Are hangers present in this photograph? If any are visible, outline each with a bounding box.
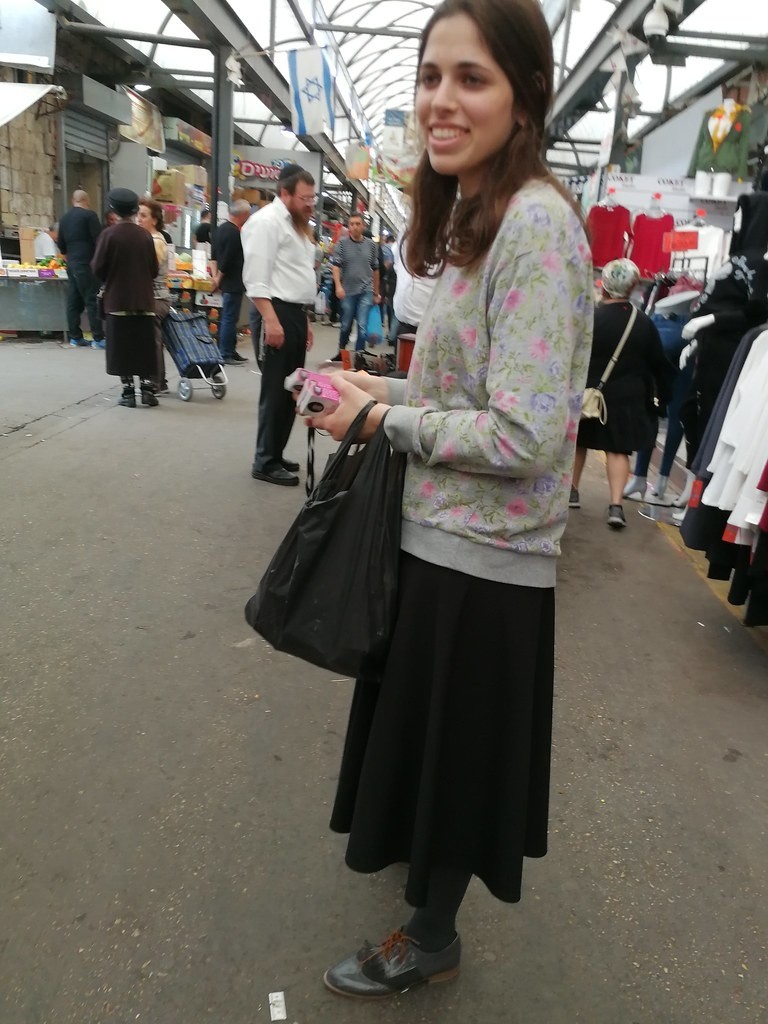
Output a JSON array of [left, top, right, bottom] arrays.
[[691, 215, 710, 227], [643, 197, 667, 216], [598, 192, 618, 207]]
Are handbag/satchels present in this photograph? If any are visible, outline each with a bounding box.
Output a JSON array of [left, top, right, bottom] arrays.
[[95, 297, 105, 321], [365, 304, 382, 344], [580, 388, 607, 426], [244, 400, 407, 686]]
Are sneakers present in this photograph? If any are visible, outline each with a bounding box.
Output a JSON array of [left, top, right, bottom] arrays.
[[70, 337, 90, 346], [567, 490, 581, 507], [608, 506, 627, 528], [92, 339, 106, 349]]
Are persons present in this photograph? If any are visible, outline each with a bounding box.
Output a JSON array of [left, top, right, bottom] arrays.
[[391, 235, 449, 365], [290, 0, 594, 1000], [33, 222, 61, 265], [104, 209, 119, 226], [321, 242, 342, 327], [568, 257, 669, 530], [211, 199, 252, 365], [240, 163, 316, 486], [362, 231, 397, 331], [89, 187, 160, 407], [308, 222, 324, 295], [622, 289, 700, 502], [134, 193, 170, 396], [326, 211, 381, 363], [57, 189, 107, 350], [193, 210, 212, 260], [672, 191, 768, 522]]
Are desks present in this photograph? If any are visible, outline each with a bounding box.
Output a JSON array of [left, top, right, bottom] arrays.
[[0, 276, 70, 343]]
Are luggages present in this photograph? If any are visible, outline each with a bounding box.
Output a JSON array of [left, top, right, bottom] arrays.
[[159, 306, 228, 401]]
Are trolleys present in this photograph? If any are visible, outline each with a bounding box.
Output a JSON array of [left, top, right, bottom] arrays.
[[162, 305, 228, 401]]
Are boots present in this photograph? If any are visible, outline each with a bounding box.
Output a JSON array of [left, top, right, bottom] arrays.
[[141, 383, 158, 406], [118, 387, 136, 407]]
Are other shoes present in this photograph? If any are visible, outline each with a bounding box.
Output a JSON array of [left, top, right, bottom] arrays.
[[332, 321, 342, 327], [223, 356, 241, 365], [321, 320, 332, 326], [140, 382, 169, 396], [330, 354, 343, 361], [232, 351, 244, 361]]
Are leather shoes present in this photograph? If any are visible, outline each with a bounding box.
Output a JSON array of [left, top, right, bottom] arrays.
[[251, 467, 298, 486], [282, 458, 299, 471], [324, 925, 461, 1000]]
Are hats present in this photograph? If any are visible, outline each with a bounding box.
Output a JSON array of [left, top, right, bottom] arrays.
[[601, 258, 640, 300]]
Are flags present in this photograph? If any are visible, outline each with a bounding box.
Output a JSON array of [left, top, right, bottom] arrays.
[[289, 46, 334, 135]]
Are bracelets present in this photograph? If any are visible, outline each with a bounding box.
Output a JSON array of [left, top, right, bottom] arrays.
[[373, 292, 379, 296]]
